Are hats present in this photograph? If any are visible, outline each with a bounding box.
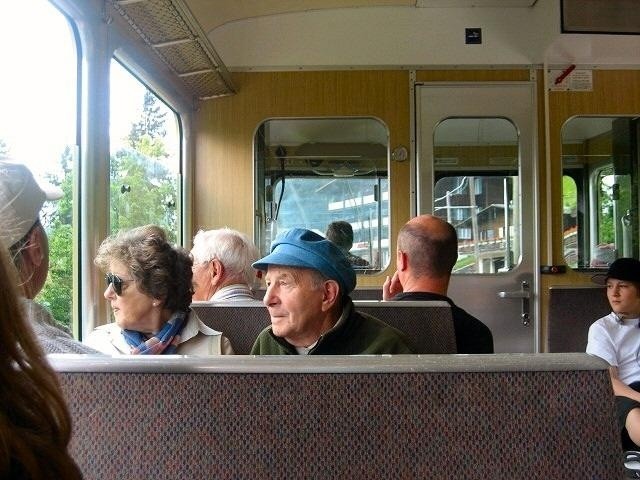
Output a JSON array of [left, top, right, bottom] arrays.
[[0, 163, 64, 247], [592, 258, 639, 285], [252, 228, 357, 294]]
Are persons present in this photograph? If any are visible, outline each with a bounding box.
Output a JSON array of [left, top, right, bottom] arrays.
[[381, 214, 496, 355], [327, 221, 368, 268], [189, 227, 264, 305], [252, 228, 417, 355], [0, 229, 84, 480], [0, 159, 103, 356], [85, 225, 234, 355], [587, 256, 640, 448]]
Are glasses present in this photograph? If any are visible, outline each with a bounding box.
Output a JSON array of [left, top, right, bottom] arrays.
[[105, 273, 135, 296]]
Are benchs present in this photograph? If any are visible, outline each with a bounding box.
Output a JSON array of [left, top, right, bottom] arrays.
[[41, 286, 640, 480]]
